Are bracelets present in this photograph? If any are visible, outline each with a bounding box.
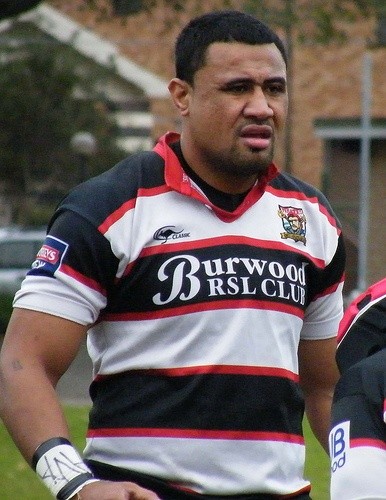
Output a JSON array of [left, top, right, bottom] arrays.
[[31, 437, 100, 500]]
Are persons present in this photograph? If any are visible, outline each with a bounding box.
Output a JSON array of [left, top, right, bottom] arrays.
[[0, 11, 386, 500]]
